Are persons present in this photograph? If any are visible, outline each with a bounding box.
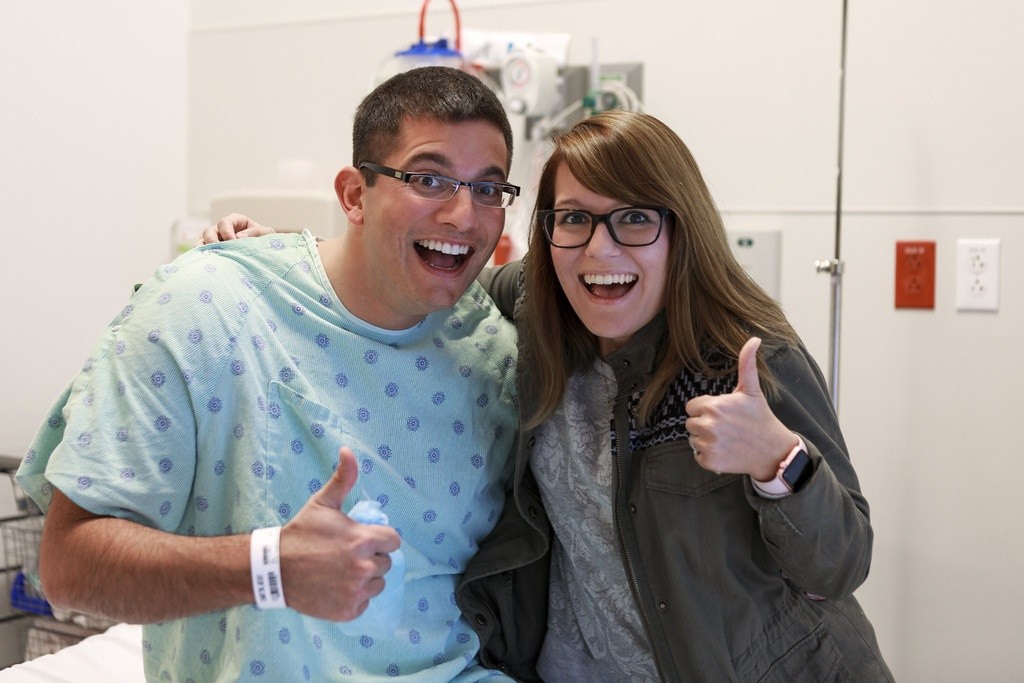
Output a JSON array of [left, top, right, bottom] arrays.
[[15, 69, 529, 683], [194, 111, 898, 683]]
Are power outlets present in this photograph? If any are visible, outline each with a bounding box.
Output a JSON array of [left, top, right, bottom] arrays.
[[959, 238, 1002, 313]]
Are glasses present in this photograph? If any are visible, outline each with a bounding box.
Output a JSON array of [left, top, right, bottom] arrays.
[[541, 205, 672, 250], [360, 160, 520, 211]]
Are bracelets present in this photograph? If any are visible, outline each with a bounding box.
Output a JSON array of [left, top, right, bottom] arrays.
[[250, 526, 288, 611]]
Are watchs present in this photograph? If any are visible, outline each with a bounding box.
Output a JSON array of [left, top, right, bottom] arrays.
[[749, 434, 812, 496]]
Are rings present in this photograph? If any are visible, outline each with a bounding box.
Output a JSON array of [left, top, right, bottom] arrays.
[[690, 438, 699, 455]]
